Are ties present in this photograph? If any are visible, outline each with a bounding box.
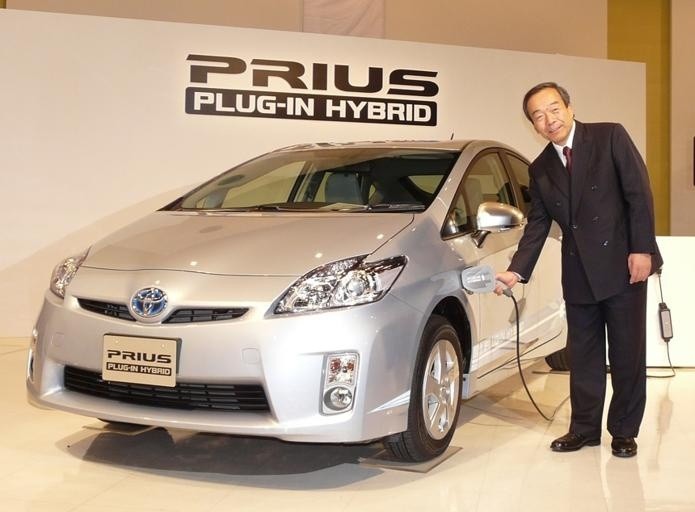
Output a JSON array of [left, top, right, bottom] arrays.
[[563, 146, 572, 175]]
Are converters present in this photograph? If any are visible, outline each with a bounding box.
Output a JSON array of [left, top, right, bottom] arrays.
[[658, 303, 673, 342]]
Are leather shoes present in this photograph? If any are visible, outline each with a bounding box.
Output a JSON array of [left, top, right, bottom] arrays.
[[550, 432, 601, 452], [611, 436, 638, 457]]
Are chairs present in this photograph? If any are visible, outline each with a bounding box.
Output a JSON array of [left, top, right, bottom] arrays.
[[324, 171, 364, 205], [367, 169, 485, 239]]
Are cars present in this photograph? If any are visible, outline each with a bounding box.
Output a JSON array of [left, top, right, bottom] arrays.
[[23, 138, 568, 464]]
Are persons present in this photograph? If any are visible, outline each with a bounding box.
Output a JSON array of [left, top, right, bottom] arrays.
[[488, 79, 666, 457]]
